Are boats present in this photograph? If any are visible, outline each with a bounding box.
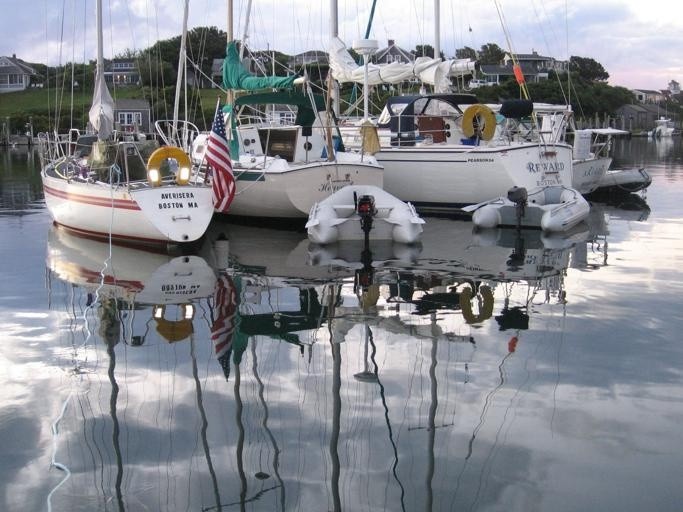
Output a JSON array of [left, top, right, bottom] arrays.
[[606, 164, 653, 195], [463, 183, 590, 235], [303, 185, 426, 246], [470, 221, 594, 256], [304, 242, 423, 268], [646, 117, 674, 136]]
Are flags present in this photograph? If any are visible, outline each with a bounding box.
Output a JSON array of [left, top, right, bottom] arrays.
[[205, 98, 236, 213], [210, 269, 236, 383]]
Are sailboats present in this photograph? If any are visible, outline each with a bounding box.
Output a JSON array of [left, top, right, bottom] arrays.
[[575, 146, 613, 190], [217, 210, 388, 509], [37, 1, 217, 255], [373, 0, 576, 216], [45, 221, 220, 508], [388, 214, 606, 512], [213, 1, 385, 222]]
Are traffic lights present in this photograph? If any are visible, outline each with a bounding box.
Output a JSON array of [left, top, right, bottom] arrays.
[[358, 196, 372, 217], [358, 269, 370, 285]]
